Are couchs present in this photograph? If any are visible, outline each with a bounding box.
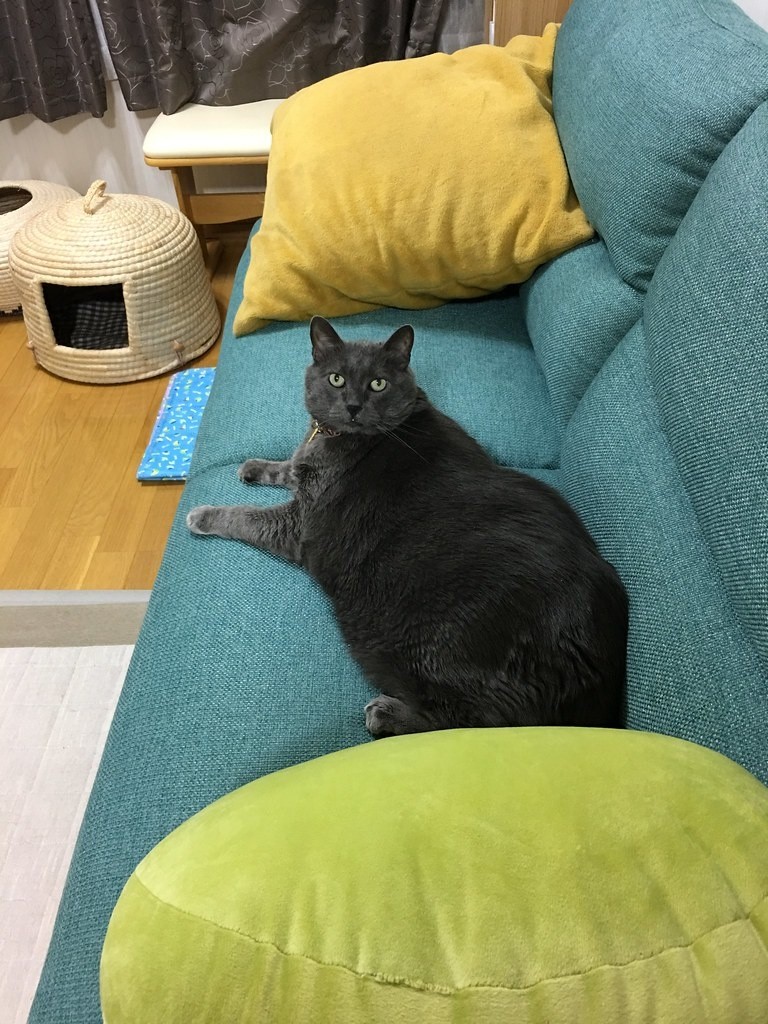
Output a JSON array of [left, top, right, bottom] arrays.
[[21, 0, 767, 1023]]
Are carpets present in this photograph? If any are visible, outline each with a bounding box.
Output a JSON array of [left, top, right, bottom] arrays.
[[2, 591, 152, 1024]]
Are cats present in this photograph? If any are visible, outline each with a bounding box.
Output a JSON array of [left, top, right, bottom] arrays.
[[185, 317, 629, 738]]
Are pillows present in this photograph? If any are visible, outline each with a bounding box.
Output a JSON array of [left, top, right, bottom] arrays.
[[230, 22, 597, 336], [94, 726, 767, 1021]]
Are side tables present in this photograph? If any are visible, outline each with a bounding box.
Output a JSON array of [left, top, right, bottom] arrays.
[[142, 97, 275, 264]]
